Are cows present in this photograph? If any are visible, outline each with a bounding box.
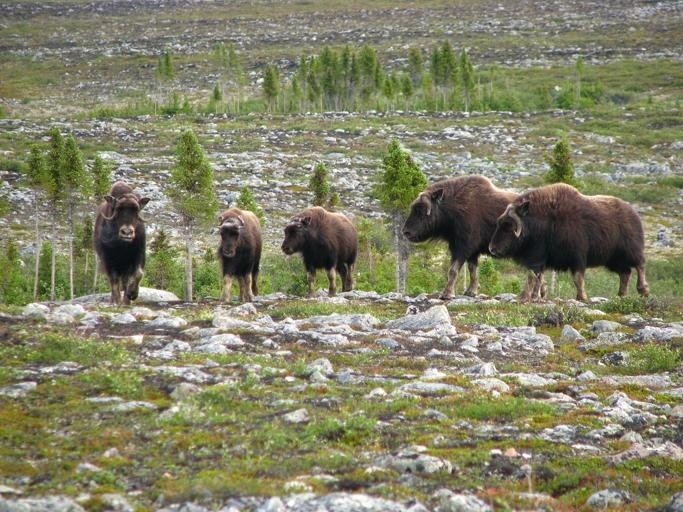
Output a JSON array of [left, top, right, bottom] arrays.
[[280, 206, 359, 300], [489, 182, 651, 302], [92, 181, 150, 312], [401, 173, 547, 302], [216, 207, 263, 305]]
